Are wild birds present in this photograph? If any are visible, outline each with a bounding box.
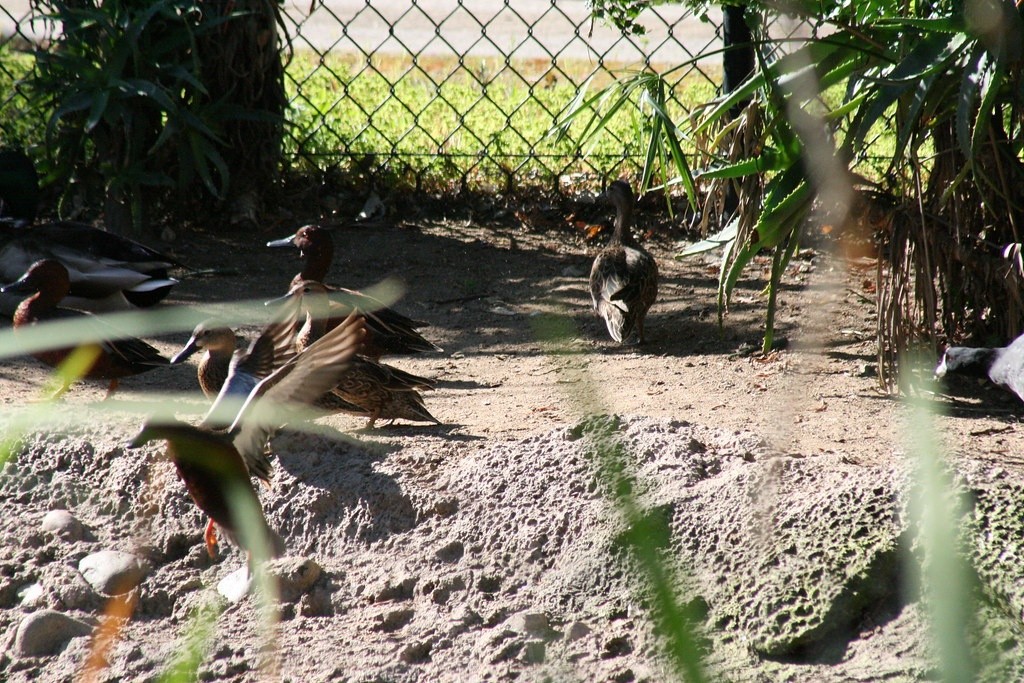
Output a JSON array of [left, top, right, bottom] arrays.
[[124, 224, 443, 582], [932, 332, 1024, 404], [0, 217, 181, 403], [587, 180, 658, 346]]
